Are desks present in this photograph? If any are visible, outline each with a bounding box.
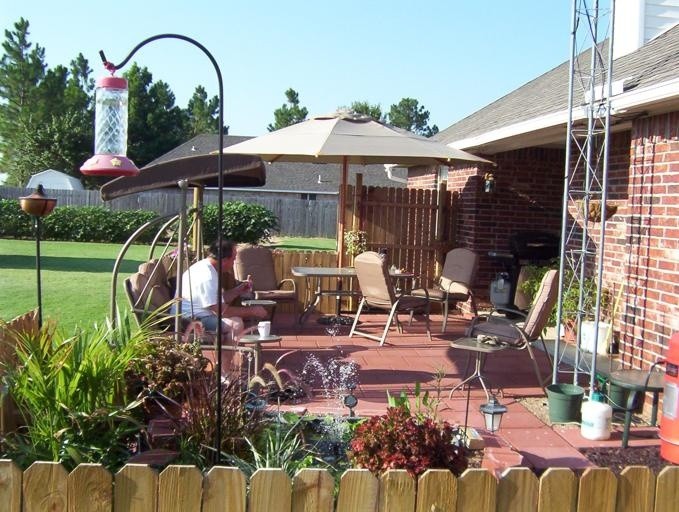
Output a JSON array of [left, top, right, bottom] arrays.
[[609, 359, 666, 451], [292, 264, 414, 328], [447, 336, 508, 402]]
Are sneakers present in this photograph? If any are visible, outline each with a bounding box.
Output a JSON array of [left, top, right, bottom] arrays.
[[220, 373, 246, 387]]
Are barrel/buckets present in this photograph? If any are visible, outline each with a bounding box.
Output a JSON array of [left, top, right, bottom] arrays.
[[580, 399, 613, 441], [578, 319, 611, 356], [659, 315, 679, 465], [543, 382, 586, 423], [243, 391, 267, 417], [605, 384, 626, 409]]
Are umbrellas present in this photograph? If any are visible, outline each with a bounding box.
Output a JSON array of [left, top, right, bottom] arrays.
[[209, 109, 497, 315]]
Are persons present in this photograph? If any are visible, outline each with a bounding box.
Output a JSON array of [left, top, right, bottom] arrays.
[[170, 239, 267, 386]]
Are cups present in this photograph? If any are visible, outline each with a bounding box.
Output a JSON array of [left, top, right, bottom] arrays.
[[257, 321, 271, 337]]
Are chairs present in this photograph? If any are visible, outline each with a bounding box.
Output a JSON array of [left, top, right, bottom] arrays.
[[409, 248, 480, 331], [462, 269, 558, 396], [348, 252, 433, 347], [130, 246, 298, 382]]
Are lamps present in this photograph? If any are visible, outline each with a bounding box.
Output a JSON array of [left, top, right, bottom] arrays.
[[478, 396, 507, 433]]
